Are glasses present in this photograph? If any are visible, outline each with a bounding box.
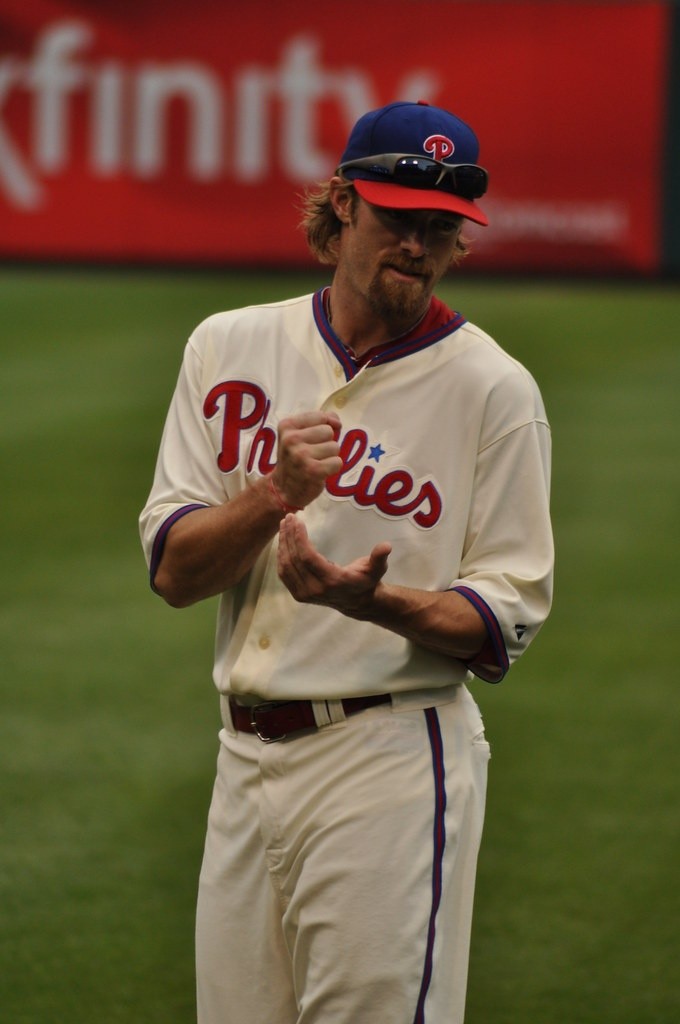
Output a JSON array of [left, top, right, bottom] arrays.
[[335, 154, 489, 200]]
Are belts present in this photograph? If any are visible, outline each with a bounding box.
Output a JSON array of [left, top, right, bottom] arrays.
[[229, 694, 392, 745]]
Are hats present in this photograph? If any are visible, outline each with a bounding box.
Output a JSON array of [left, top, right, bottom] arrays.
[[340, 99, 491, 227]]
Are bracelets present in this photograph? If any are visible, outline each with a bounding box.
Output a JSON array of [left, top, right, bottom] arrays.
[[269, 471, 304, 514]]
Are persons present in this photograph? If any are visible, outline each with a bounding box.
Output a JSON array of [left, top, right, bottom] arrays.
[[136, 98, 557, 1024]]
